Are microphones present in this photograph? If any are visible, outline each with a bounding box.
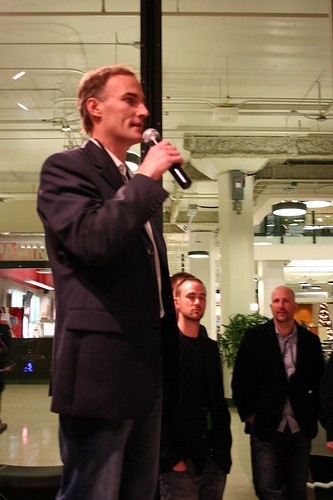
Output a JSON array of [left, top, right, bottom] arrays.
[[142, 128, 192, 189]]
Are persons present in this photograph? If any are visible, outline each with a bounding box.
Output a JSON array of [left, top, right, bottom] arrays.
[[37, 65, 186, 498], [168, 272, 193, 312], [320, 352, 333, 453], [156, 276, 234, 500], [230, 286, 326, 500], [1, 333, 9, 434]]
